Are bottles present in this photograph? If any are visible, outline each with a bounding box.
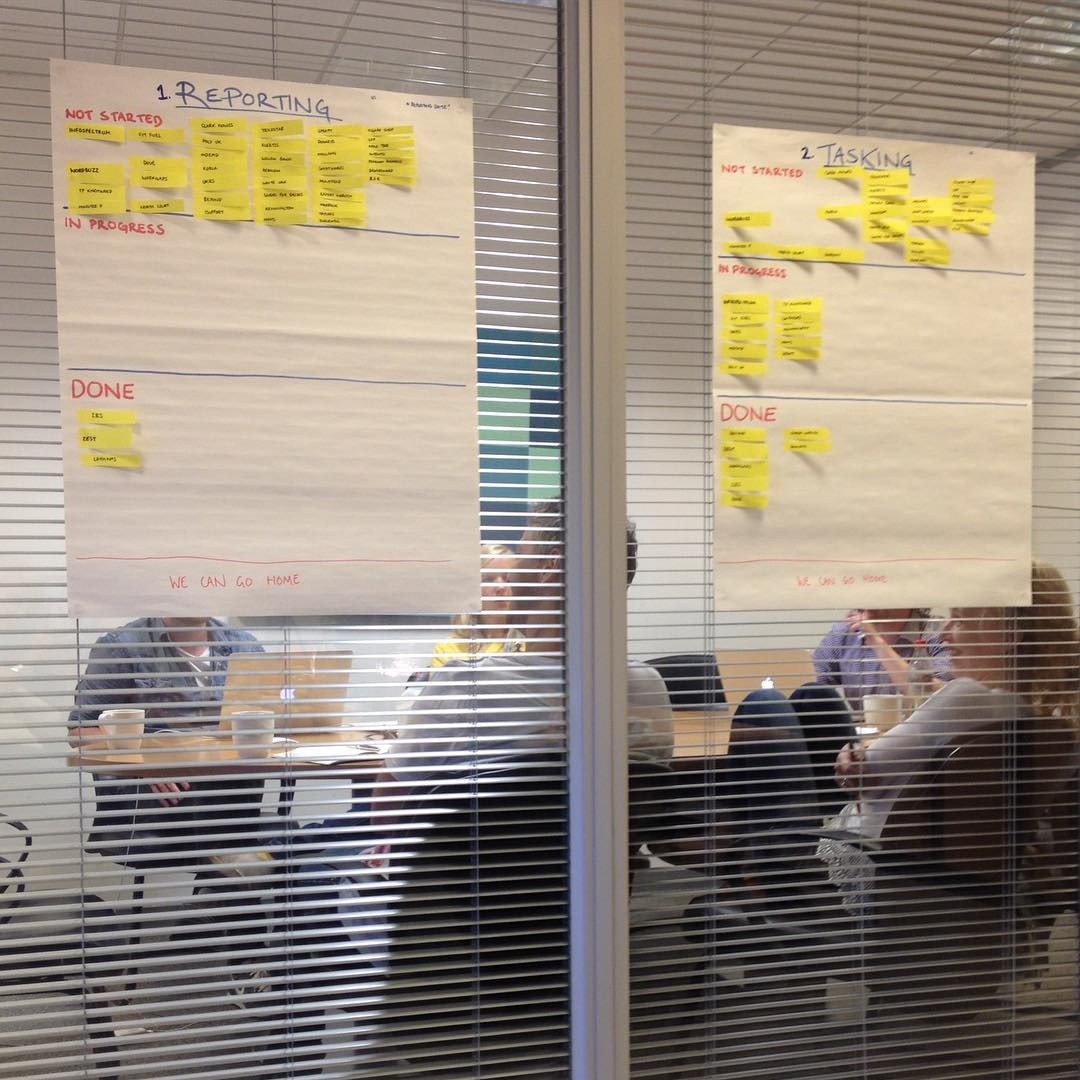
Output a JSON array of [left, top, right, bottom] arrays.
[[907, 640, 933, 716]]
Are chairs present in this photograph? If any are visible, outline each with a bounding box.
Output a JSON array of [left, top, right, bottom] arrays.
[[677, 714, 1080, 1079], [0, 813, 128, 1080], [644, 656, 728, 869], [311, 759, 715, 1080]]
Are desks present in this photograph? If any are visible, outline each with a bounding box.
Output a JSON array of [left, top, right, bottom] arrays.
[[68, 713, 879, 1006]]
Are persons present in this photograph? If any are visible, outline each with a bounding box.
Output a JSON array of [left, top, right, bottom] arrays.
[[66, 493, 1079, 1030]]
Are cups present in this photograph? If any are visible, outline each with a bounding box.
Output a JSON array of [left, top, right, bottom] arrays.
[[863, 695, 903, 731], [98, 709, 146, 750], [232, 710, 274, 760]]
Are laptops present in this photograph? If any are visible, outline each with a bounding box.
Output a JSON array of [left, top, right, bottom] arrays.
[[188, 650, 352, 738], [713, 648, 818, 715]]
